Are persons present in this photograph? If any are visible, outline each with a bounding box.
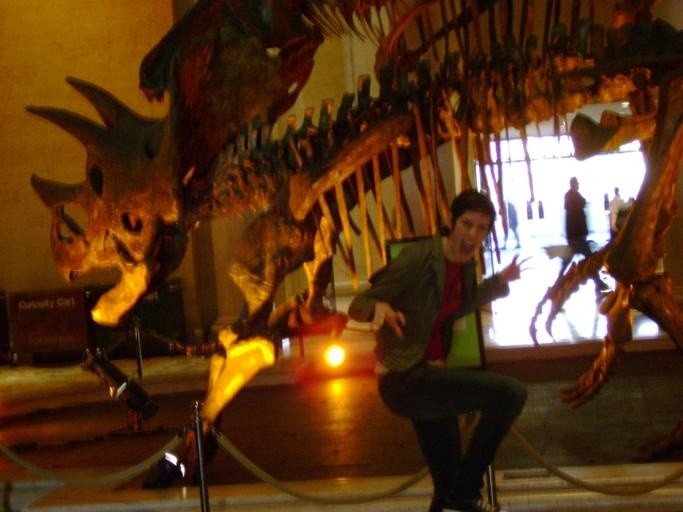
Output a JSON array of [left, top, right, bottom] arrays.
[[348, 186, 532, 512], [495, 193, 520, 250], [608, 186, 624, 228], [562, 175, 589, 244]]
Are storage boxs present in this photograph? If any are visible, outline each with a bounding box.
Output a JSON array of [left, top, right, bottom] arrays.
[[85, 277, 185, 359], [7, 280, 97, 365]]
